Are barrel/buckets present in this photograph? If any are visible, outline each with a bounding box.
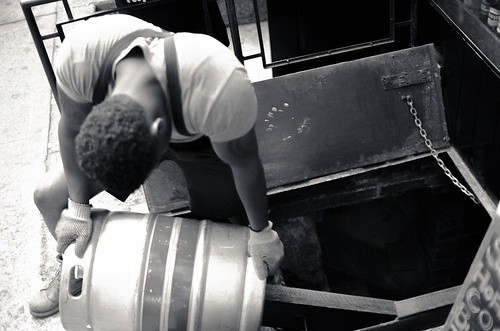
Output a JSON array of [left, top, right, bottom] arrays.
[[59, 208, 267, 331]]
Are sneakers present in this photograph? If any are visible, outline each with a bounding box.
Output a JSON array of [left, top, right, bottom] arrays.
[[29, 254, 82, 317]]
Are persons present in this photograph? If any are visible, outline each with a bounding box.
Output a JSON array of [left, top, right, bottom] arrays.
[[29, 14, 284, 317]]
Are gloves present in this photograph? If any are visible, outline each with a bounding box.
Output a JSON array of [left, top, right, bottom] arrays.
[[248, 221, 285, 280], [54, 198, 93, 258]]
